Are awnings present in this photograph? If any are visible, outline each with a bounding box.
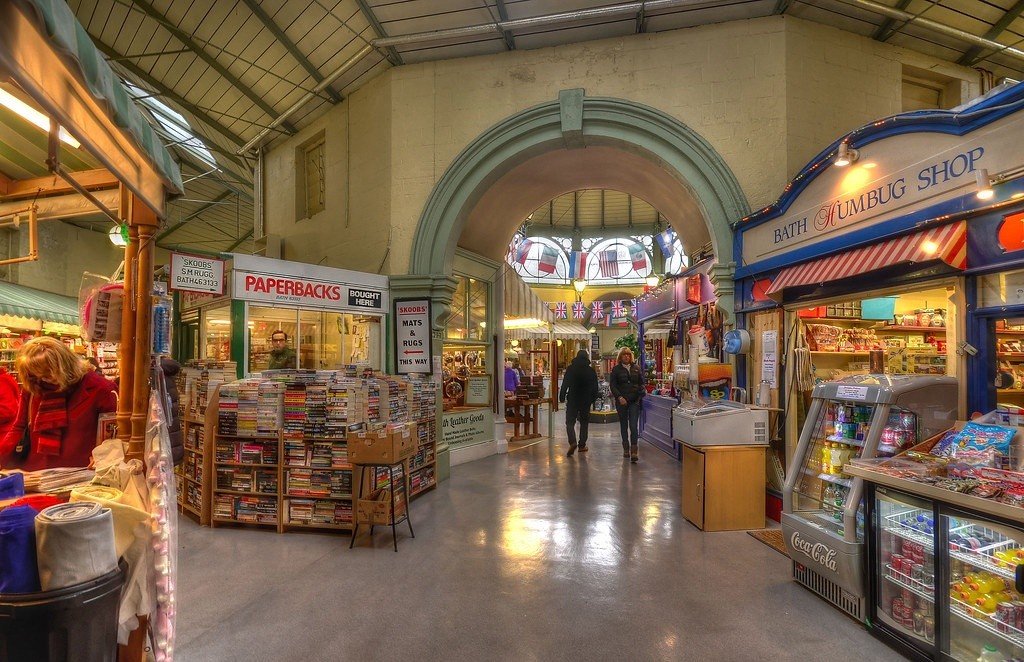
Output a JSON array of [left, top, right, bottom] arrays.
[[503, 321, 592, 385], [0, 280, 79, 326]]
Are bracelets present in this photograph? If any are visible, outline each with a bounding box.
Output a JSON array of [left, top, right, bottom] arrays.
[[620, 397, 623, 399]]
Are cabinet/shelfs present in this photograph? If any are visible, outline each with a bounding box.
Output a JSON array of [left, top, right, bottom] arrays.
[[681, 444, 766, 531], [1, 323, 488, 533], [878, 502, 1024, 662], [799, 318, 1024, 393]]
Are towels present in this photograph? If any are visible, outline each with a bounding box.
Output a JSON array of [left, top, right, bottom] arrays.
[[792, 344, 815, 393]]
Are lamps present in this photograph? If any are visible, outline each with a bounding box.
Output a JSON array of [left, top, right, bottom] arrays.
[[0, 88, 85, 151], [835, 144, 860, 166], [108, 223, 130, 249], [976, 169, 999, 200]]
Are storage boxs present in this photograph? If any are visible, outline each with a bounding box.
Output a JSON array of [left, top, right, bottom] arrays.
[[884, 336, 946, 353], [357, 489, 406, 526], [827, 300, 861, 319], [860, 296, 901, 319], [798, 306, 827, 318], [347, 421, 418, 464]]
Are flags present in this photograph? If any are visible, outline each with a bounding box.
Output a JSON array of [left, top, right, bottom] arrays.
[[572, 302, 584, 318], [611, 300, 623, 318], [592, 301, 604, 318], [569, 252, 587, 278], [538, 246, 559, 274], [599, 250, 619, 277], [629, 242, 646, 271], [654, 228, 674, 259], [516, 237, 532, 264], [631, 299, 637, 316], [556, 302, 566, 319]]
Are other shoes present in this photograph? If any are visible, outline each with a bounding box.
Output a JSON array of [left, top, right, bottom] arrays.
[[565, 442, 578, 456], [577, 446, 589, 452]]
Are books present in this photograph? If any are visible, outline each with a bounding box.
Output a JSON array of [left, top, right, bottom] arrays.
[[179, 360, 436, 525]]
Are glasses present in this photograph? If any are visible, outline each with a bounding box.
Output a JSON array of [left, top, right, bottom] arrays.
[[273, 339, 286, 343]]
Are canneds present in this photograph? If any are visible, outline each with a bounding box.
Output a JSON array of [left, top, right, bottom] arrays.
[[995, 600, 1024, 634], [889, 539, 973, 642], [880, 409, 917, 450]]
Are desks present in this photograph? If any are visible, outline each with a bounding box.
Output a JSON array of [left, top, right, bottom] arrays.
[[506, 398, 552, 442]]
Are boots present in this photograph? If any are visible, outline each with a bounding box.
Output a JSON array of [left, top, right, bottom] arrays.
[[631, 445, 640, 462], [621, 441, 631, 458]]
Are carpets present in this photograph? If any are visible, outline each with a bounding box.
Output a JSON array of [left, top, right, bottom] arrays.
[[746, 528, 788, 557]]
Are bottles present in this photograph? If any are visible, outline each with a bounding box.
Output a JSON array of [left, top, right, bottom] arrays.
[[903, 514, 1024, 619], [822, 441, 865, 532], [977, 643, 1005, 662]]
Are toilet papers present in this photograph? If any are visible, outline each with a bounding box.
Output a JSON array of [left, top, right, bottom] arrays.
[[721, 329, 751, 355]]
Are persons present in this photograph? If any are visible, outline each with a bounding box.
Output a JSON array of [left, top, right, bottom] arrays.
[[268, 331, 297, 369], [610, 347, 646, 462], [151, 358, 184, 467], [0, 351, 20, 444], [506, 361, 524, 416], [560, 350, 598, 455], [0, 337, 119, 471]]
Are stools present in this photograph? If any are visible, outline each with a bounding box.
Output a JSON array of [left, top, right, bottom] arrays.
[[350, 458, 417, 551]]
